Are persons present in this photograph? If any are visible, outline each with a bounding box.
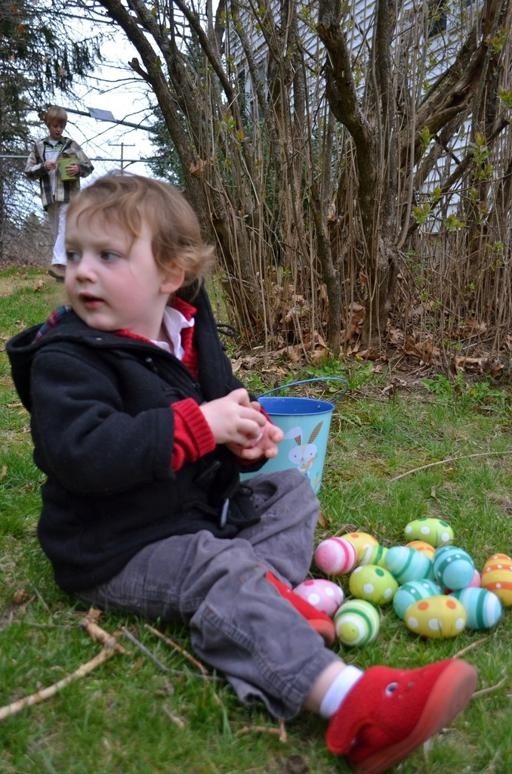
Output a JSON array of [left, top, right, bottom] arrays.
[[25, 106, 94, 282], [5, 166, 481, 774]]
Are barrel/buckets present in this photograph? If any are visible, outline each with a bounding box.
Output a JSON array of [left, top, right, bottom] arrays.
[[239, 378, 351, 501], [58, 158, 78, 180]]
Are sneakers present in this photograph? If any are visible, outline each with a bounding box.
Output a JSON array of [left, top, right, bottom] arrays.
[[48, 265, 64, 280], [265, 572, 336, 646], [326, 658, 477, 773]]
